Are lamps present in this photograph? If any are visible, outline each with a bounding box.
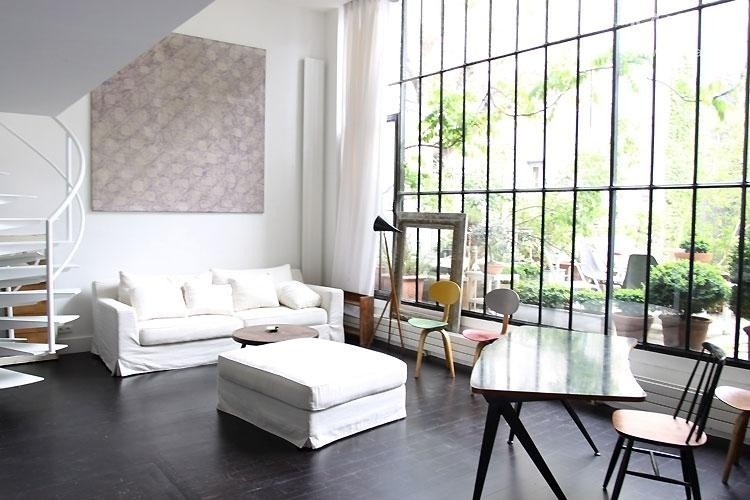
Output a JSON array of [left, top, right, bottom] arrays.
[[366, 217, 407, 357]]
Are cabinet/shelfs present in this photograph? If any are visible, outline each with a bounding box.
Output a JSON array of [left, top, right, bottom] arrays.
[[343, 291, 375, 348]]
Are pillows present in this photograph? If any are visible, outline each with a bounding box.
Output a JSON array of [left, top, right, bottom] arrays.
[[181, 283, 235, 316], [117, 270, 168, 307], [279, 279, 320, 309], [228, 276, 279, 311], [130, 283, 187, 320], [160, 270, 212, 295], [208, 268, 248, 285], [249, 263, 292, 292]]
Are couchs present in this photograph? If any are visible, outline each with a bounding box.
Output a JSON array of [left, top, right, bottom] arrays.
[[92, 264, 344, 378]]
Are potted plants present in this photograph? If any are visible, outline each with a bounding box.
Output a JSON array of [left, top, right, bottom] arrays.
[[540, 287, 571, 328], [572, 288, 606, 333], [512, 284, 540, 324], [674, 239, 712, 263], [646, 258, 732, 352], [609, 288, 654, 339], [728, 218, 749, 363]]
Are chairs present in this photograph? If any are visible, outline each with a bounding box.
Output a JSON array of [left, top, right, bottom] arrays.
[[408, 280, 460, 378], [462, 289, 520, 396], [715, 326, 750, 485], [602, 342, 726, 500]]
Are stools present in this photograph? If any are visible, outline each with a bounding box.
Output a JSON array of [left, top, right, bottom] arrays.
[[219, 337, 407, 455]]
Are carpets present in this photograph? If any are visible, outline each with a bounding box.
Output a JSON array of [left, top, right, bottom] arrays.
[[0, 367, 44, 390]]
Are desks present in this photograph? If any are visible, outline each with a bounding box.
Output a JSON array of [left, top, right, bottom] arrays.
[[470, 326, 647, 500]]
